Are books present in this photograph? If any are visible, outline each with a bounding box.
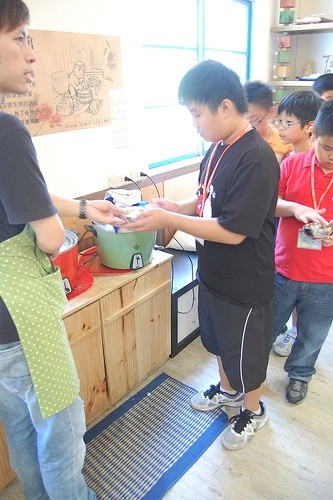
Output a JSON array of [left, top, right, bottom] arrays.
[[298, 16, 333, 25]]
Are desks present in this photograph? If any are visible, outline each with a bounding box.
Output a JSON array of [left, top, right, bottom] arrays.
[[155, 248, 202, 359], [0, 249, 175, 490]]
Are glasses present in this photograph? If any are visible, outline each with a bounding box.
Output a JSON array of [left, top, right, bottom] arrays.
[[272, 115, 310, 129], [247, 107, 271, 127]]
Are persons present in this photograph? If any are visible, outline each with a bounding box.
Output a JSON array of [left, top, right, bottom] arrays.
[[123, 60, 333, 452], [1, 0, 126, 499]]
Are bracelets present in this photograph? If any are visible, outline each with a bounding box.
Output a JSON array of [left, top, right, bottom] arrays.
[[79, 198, 87, 219]]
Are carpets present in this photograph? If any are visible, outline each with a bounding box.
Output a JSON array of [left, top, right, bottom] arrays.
[[82, 372, 230, 500]]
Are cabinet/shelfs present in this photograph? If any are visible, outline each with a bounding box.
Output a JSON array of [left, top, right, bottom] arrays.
[[268, 22, 333, 87]]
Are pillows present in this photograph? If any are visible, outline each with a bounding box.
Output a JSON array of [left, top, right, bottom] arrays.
[[165, 194, 211, 252]]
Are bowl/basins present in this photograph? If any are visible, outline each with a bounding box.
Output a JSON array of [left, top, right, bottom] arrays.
[[303, 222, 333, 240], [122, 206, 144, 222]]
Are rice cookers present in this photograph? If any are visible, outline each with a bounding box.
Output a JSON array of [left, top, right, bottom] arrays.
[[84, 220, 157, 269], [52, 229, 78, 294]]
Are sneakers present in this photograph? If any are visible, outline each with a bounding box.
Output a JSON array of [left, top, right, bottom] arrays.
[[285, 378, 308, 404], [190, 381, 245, 411], [221, 401, 268, 451], [274, 327, 298, 357]]
